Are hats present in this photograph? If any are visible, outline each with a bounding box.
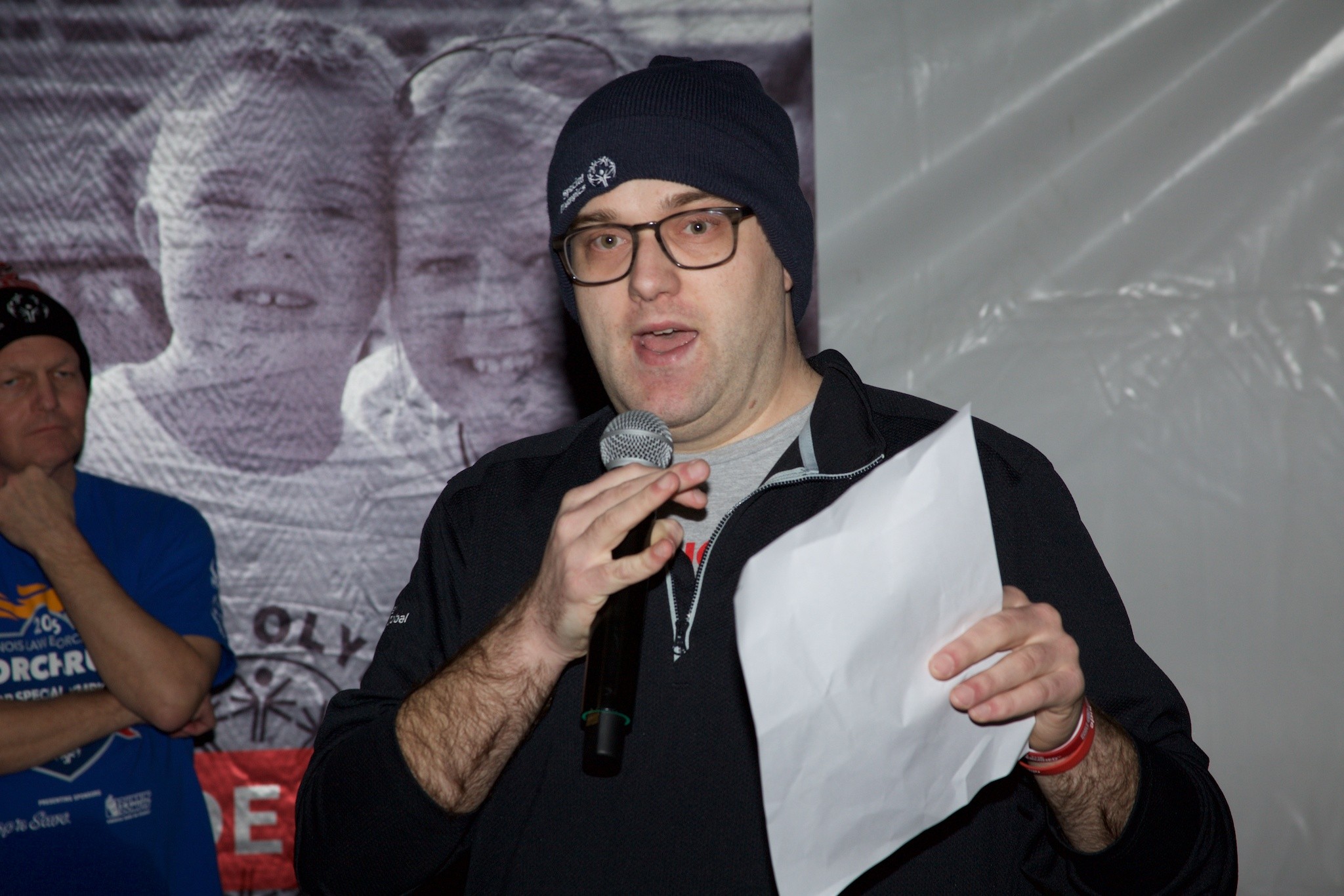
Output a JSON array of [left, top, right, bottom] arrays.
[[0, 274, 91, 390], [545, 54, 814, 327]]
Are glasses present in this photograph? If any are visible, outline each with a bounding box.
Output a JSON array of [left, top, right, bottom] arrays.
[[552, 205, 754, 287], [393, 31, 616, 117]]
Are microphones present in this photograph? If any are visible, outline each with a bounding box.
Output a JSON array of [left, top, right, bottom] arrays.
[[579, 410, 673, 775]]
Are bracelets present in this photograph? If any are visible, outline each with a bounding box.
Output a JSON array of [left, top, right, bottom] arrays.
[[1018, 697, 1097, 775], [1025, 701, 1091, 762]]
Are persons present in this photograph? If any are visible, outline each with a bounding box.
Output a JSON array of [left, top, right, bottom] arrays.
[[342, 31, 637, 481], [293, 55, 1240, 896], [0, 280, 239, 896], [76, 12, 445, 752]]
